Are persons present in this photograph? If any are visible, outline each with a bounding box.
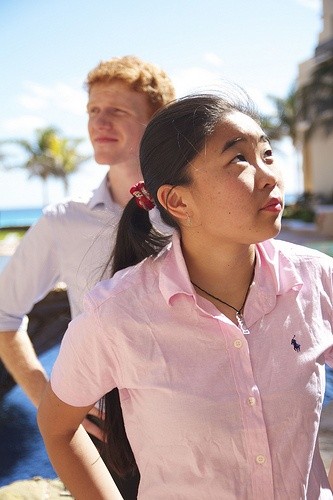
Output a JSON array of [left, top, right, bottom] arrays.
[[32, 93, 333, 500], [0, 57, 176, 500]]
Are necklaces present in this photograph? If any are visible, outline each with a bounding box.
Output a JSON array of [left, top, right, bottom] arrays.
[[190, 251, 256, 335]]
